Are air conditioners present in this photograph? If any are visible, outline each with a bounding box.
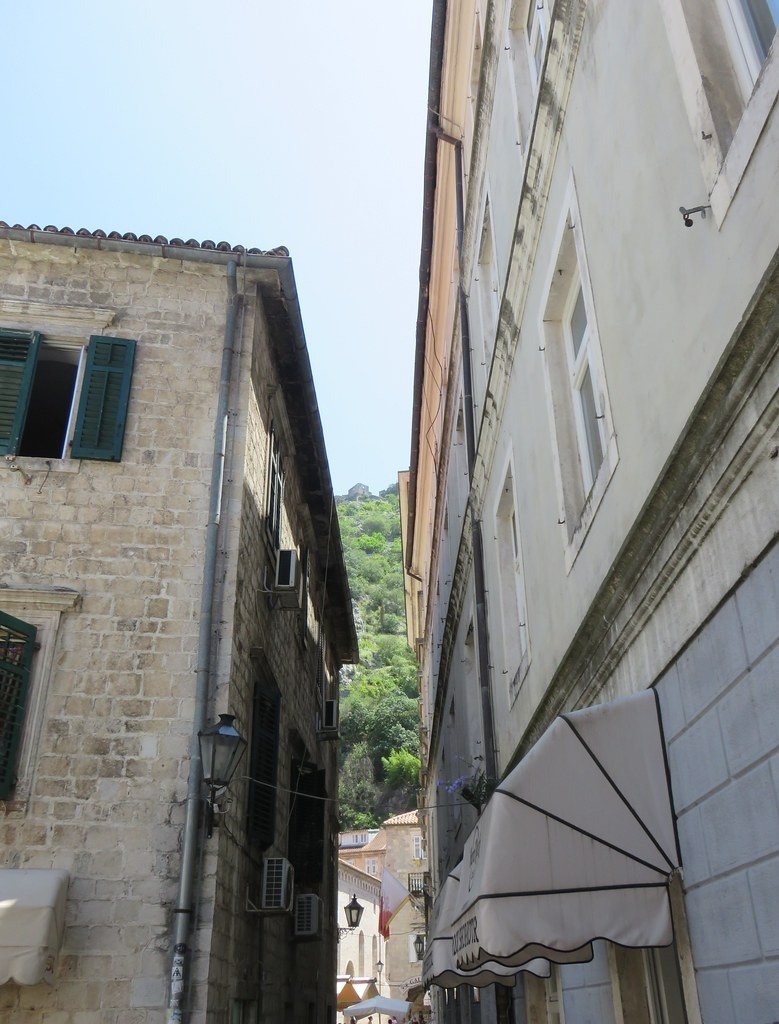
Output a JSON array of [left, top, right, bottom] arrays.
[[323, 699, 339, 743], [295, 892, 324, 937], [276, 549, 307, 611], [262, 857, 295, 914]]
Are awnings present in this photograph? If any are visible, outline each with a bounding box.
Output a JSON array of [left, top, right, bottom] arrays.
[[452, 688, 686, 972], [422, 864, 552, 989]]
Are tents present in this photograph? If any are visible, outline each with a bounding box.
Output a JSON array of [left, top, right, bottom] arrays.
[[343, 995, 413, 1024]]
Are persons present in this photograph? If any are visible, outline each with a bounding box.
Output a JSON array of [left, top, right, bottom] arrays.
[[408, 1016, 427, 1024], [349, 1016, 355, 1024], [368, 1016, 373, 1024], [388, 1016, 398, 1024]]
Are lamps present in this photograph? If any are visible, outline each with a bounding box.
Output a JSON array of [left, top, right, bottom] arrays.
[[338, 892, 364, 932], [413, 935, 424, 961], [376, 961, 384, 973], [198, 714, 249, 838]]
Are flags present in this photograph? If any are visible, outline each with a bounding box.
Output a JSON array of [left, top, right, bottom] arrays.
[[378, 866, 410, 941]]
